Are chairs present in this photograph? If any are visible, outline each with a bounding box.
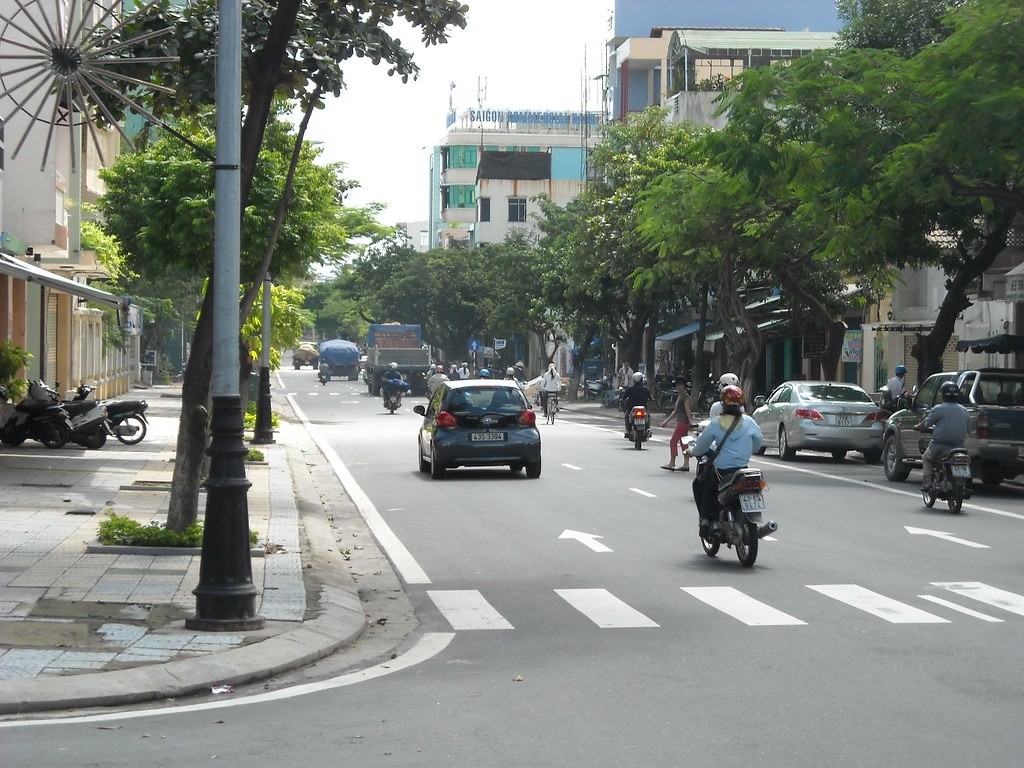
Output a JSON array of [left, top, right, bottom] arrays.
[[491, 391, 510, 405], [996, 392, 1014, 406]]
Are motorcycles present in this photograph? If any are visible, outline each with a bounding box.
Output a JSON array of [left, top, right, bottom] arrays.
[[320, 371, 327, 385], [384, 387, 403, 414], [918, 426, 975, 514], [679, 423, 779, 568], [617, 387, 653, 451]]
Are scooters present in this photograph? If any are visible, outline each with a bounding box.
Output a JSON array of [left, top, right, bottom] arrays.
[[2, 378, 150, 450]]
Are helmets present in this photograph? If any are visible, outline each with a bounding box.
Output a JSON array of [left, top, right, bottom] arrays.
[[322, 359, 325, 362], [719, 385, 744, 405], [437, 366, 442, 372], [391, 362, 397, 368], [431, 364, 435, 368], [719, 373, 738, 391], [941, 381, 958, 397], [895, 366, 906, 374]]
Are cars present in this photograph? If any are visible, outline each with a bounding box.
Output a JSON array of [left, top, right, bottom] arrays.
[[751, 380, 881, 464], [413, 378, 541, 479]]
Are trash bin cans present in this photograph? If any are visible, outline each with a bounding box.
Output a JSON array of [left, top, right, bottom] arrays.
[[248, 371, 261, 402], [143, 370, 153, 385]]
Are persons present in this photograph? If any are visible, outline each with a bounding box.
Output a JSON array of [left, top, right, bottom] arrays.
[[503, 361, 528, 384], [685, 373, 763, 536], [381, 362, 403, 406], [479, 367, 489, 379], [618, 358, 634, 399], [426, 362, 470, 398], [537, 361, 561, 417], [913, 381, 972, 492], [660, 375, 693, 471], [318, 359, 329, 382], [623, 372, 652, 434], [887, 365, 908, 401]]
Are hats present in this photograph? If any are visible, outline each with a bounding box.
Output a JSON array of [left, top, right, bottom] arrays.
[[672, 375, 686, 383], [507, 368, 514, 375], [480, 370, 489, 376], [632, 372, 645, 382]]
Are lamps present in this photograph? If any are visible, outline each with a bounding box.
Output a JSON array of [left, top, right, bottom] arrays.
[[887, 309, 892, 321]]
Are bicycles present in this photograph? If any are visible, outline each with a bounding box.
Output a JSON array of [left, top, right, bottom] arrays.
[[545, 393, 557, 425]]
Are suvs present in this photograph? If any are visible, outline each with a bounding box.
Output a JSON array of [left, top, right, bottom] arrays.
[[882, 367, 1024, 487]]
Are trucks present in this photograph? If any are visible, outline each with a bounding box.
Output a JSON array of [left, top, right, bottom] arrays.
[[363, 323, 429, 396], [319, 339, 360, 381], [292, 342, 319, 370]]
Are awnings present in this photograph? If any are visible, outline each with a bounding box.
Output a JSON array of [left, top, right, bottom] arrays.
[[955, 334, 1024, 354], [655, 315, 807, 341]]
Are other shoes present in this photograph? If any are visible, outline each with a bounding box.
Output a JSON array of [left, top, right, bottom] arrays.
[[699, 525, 709, 537], [921, 475, 934, 490], [675, 466, 689, 471], [661, 463, 675, 471]]
